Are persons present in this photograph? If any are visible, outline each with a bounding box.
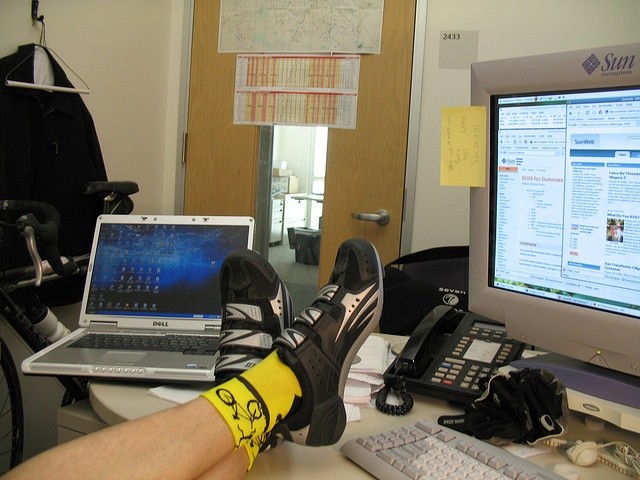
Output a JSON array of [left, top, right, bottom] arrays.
[[0, 237, 385, 480], [607, 219, 623, 242]]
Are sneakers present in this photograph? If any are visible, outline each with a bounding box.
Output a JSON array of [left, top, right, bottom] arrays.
[[214, 249, 295, 453], [272, 239, 384, 446]]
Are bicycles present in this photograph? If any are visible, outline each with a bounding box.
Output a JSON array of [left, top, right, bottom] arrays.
[[1, 180, 139, 472]]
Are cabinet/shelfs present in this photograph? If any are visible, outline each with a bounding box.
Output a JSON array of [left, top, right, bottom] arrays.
[[283, 191, 309, 246], [269, 198, 285, 247], [53, 400, 113, 447]]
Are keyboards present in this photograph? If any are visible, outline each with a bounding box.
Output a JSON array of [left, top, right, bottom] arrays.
[[341, 420, 570, 480]]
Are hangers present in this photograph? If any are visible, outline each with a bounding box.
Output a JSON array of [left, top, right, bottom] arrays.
[[2, 15, 94, 100]]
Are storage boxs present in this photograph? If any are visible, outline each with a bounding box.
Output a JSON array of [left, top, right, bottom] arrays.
[[288, 176, 301, 194]]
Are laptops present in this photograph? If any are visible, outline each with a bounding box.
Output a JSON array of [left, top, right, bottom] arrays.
[[21, 214, 255, 382]]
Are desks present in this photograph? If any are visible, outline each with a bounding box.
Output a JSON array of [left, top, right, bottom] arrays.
[[83, 328, 639, 479]]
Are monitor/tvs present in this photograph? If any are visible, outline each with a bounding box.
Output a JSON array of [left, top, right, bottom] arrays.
[[469, 44, 640, 434]]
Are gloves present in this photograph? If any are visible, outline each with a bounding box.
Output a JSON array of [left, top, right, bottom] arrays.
[[438, 368, 571, 446]]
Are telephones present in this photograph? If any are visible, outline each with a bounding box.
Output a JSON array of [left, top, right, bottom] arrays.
[[376, 304, 526, 416]]
